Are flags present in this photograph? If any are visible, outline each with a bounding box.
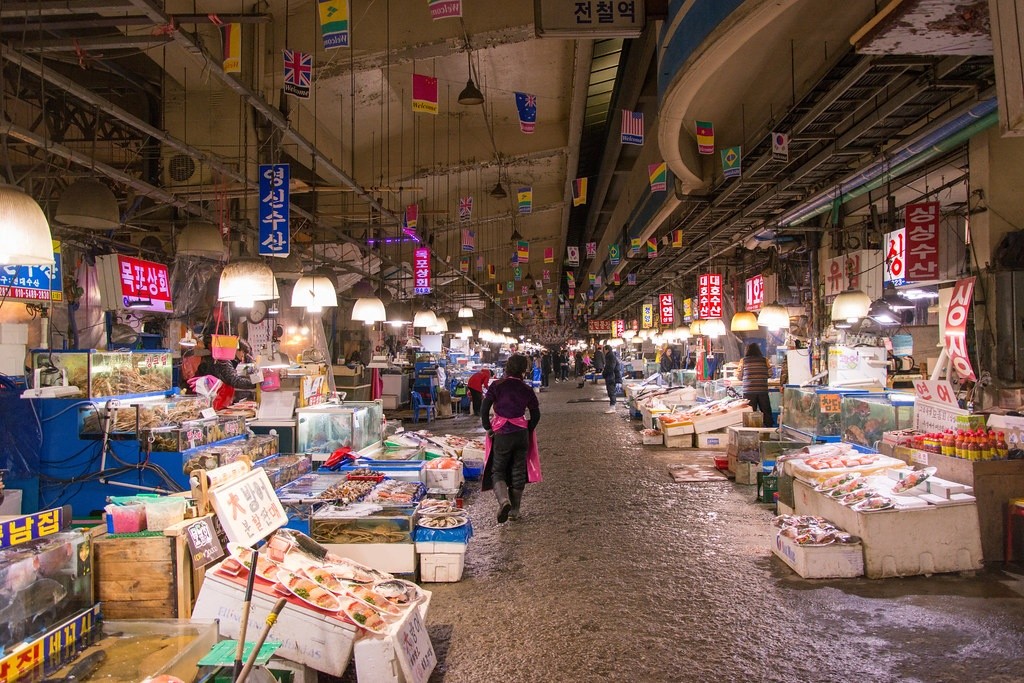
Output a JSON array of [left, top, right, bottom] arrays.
[[282, 50, 312, 100], [621, 109, 644, 146], [427, 0, 463, 20], [412, 74, 438, 114], [402, 121, 787, 348], [515, 91, 536, 134], [217, 22, 242, 74], [319, 0, 349, 49]]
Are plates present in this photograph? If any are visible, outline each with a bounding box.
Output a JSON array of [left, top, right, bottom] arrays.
[[417, 505, 468, 528], [220, 536, 424, 634], [774, 467, 937, 547]]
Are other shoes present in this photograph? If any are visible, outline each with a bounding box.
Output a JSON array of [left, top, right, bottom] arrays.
[[605, 409, 616, 413], [542, 386, 549, 389], [565, 377, 568, 381]]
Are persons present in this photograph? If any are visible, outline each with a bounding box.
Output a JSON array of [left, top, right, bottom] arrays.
[[779, 339, 801, 393], [213, 338, 256, 389], [510, 344, 570, 388], [467, 369, 493, 416], [481, 355, 541, 523], [583, 354, 591, 372], [574, 354, 584, 389], [603, 345, 622, 414], [661, 348, 675, 371], [737, 343, 773, 427], [594, 347, 605, 372], [682, 357, 695, 369]]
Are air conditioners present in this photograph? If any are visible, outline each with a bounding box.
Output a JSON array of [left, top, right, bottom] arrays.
[[131, 231, 175, 260], [163, 149, 216, 186]]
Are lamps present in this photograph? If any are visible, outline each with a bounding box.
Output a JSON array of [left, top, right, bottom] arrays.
[[291, 154, 339, 307], [758, 232, 790, 329], [607, 243, 726, 346], [413, 206, 518, 344], [509, 217, 524, 242], [457, 49, 484, 105], [868, 291, 916, 325], [526, 265, 543, 320], [351, 224, 388, 323], [831, 231, 871, 320], [730, 246, 760, 331], [55, 103, 120, 229], [491, 160, 507, 198], [1, 183, 55, 264], [217, 94, 279, 301], [365, 322, 394, 369], [176, 158, 226, 256]]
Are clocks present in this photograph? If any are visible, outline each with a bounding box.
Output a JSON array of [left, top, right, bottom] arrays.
[[249, 301, 268, 324]]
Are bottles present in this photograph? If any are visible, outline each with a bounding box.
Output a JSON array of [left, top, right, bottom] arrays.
[[337, 354, 346, 365], [907, 429, 1023, 461]]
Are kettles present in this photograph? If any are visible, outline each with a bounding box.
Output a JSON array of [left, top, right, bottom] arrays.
[[899, 355, 915, 373], [886, 356, 895, 374]]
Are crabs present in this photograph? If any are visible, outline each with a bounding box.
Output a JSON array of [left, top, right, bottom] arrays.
[[154, 433, 207, 452], [313, 519, 411, 543], [83, 399, 209, 432], [68, 367, 170, 398], [320, 479, 417, 504]]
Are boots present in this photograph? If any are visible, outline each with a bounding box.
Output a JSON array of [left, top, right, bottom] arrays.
[[508, 488, 522, 520], [494, 480, 511, 523]]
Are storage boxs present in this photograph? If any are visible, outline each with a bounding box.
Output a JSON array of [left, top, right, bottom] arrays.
[[191, 565, 360, 678], [639, 401, 753, 448], [109, 506, 147, 532], [783, 384, 869, 437], [714, 454, 729, 469], [880, 442, 1024, 564], [417, 541, 467, 553], [729, 426, 779, 446], [771, 524, 866, 579], [340, 433, 487, 496], [274, 472, 421, 574], [353, 636, 402, 683], [623, 362, 698, 415], [294, 399, 384, 457], [725, 454, 738, 473], [146, 503, 185, 531], [757, 471, 777, 502], [792, 479, 983, 575], [30, 346, 312, 492], [419, 552, 465, 582], [758, 441, 809, 460], [840, 393, 917, 455]]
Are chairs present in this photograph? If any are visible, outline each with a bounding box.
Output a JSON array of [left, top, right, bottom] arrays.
[[410, 390, 435, 425]]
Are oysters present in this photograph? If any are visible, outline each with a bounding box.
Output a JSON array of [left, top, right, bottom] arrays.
[[421, 505, 465, 527]]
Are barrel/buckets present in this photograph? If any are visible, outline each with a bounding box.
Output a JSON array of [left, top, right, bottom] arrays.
[[210, 334, 238, 360], [259, 368, 281, 391]]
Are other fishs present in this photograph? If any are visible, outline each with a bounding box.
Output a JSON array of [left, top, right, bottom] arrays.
[[307, 431, 351, 452], [0, 538, 74, 645], [847, 402, 888, 446], [788, 394, 840, 434]]
